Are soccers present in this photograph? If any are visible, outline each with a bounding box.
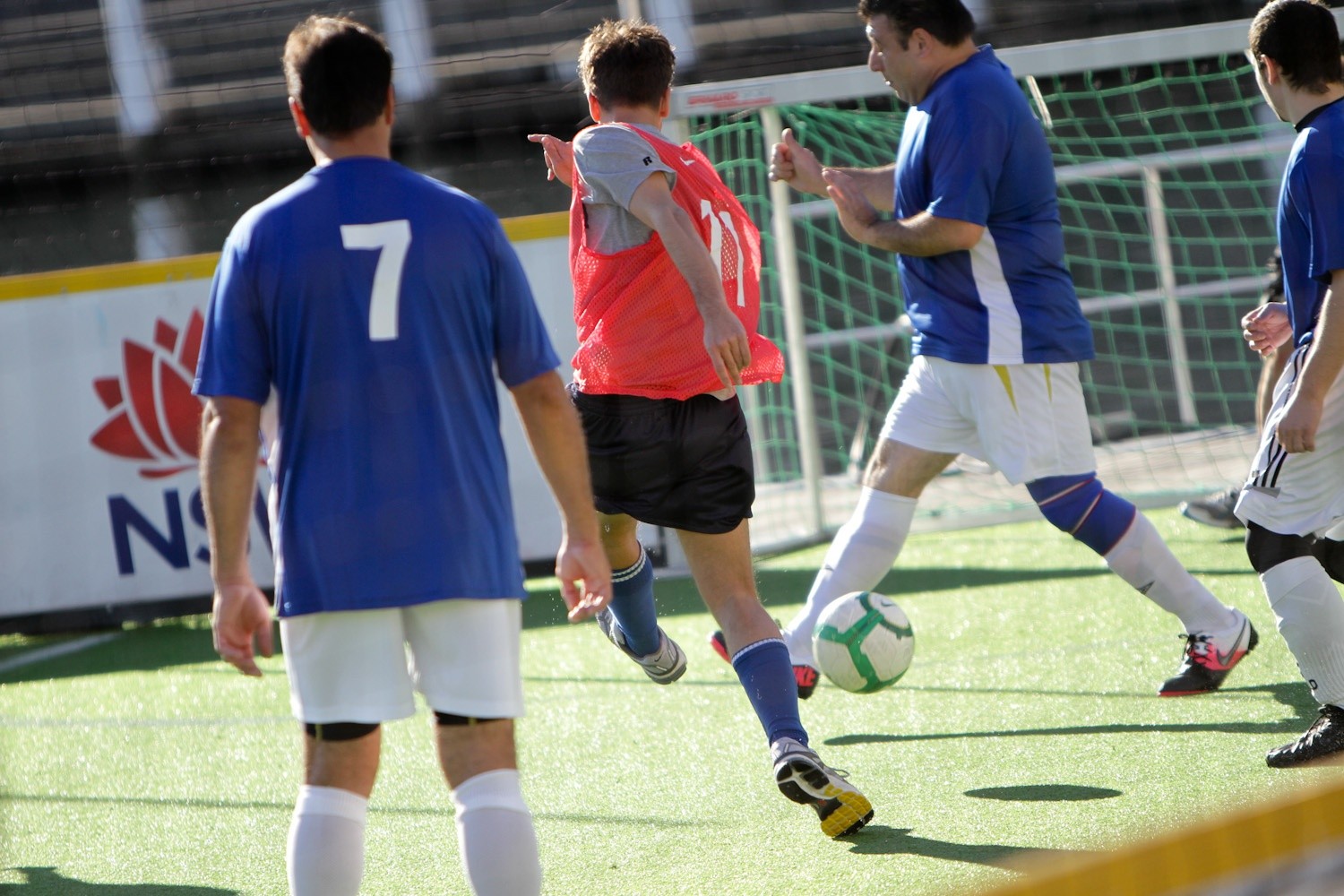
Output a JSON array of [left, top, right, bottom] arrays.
[[811, 591, 916, 696]]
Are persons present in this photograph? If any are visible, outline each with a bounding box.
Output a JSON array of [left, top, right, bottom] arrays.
[[711, 0, 1260, 702], [192, 17, 611, 896], [527, 21, 876, 840], [1179, 1, 1344, 766]]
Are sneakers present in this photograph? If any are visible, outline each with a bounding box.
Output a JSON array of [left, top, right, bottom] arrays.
[[1158, 606, 1260, 696], [1266, 704, 1344, 767], [771, 736, 874, 839], [711, 619, 820, 700], [1178, 475, 1250, 528], [595, 607, 688, 685]]
